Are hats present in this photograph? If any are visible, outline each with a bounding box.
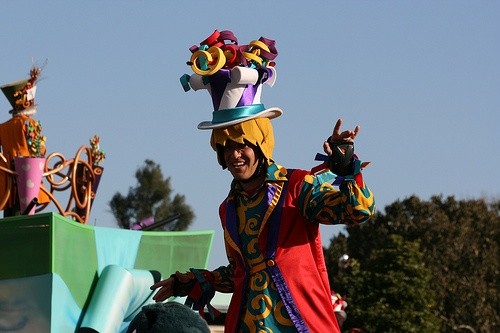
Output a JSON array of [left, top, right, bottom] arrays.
[[189, 65, 282, 129], [209, 118, 274, 169], [1, 58, 49, 116]]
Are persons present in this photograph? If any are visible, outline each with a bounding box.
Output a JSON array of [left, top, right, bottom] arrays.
[[1, 65, 54, 218], [150, 29, 376, 333]]
